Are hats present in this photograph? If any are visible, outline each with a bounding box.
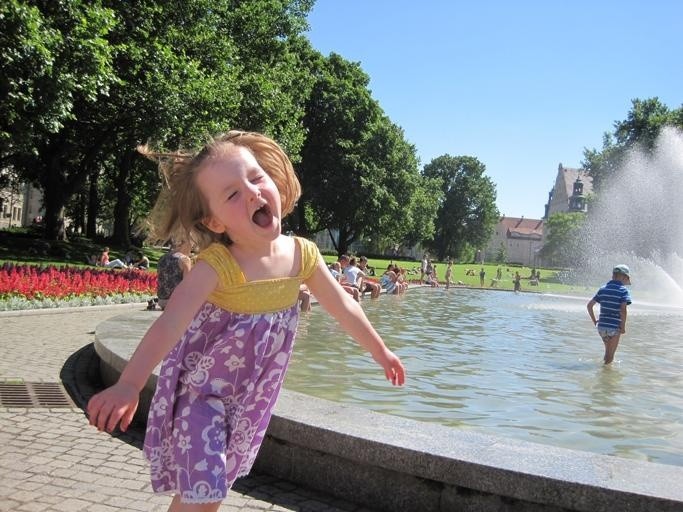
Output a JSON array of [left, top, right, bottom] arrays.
[[613, 265, 631, 285]]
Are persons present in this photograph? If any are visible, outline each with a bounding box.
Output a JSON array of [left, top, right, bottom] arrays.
[[586, 265, 632, 365], [467, 265, 541, 291], [83, 128, 406, 512], [408, 253, 453, 289], [157, 244, 192, 311], [298, 254, 409, 309], [100, 246, 149, 271]]
